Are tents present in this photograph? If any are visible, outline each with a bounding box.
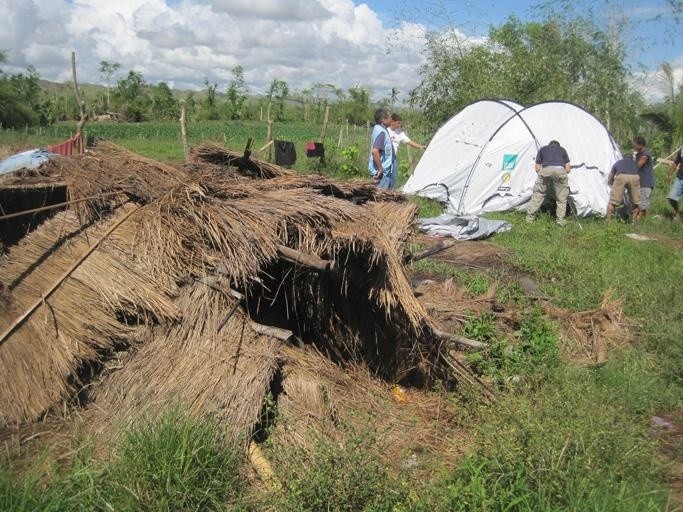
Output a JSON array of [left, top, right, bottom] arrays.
[[456, 100, 624, 220], [403, 100, 525, 211]]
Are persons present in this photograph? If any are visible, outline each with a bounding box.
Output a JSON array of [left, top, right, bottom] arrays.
[[636, 137, 654, 216], [525, 141, 571, 224], [606, 153, 640, 226], [387, 113, 427, 188], [369, 108, 395, 190], [666, 144, 683, 221]]
